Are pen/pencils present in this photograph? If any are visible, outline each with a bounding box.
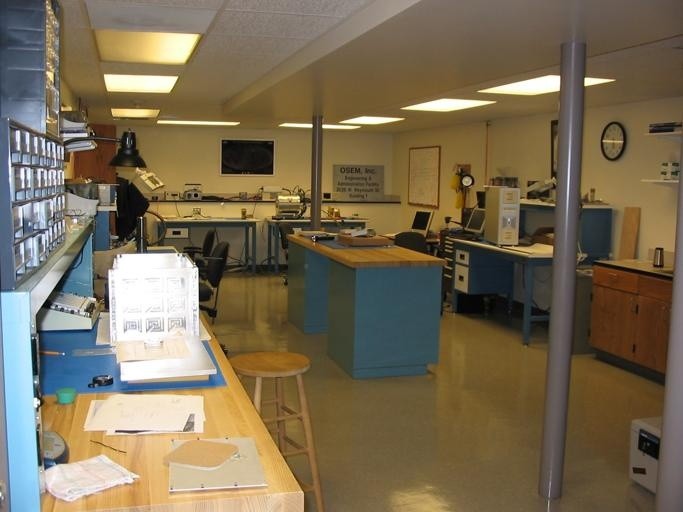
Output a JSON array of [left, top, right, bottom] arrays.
[[40, 351, 65, 356]]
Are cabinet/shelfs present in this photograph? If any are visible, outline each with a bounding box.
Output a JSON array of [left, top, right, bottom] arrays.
[[640, 132, 682, 184], [588, 261, 673, 386], [451, 243, 513, 295], [448, 200, 612, 346]]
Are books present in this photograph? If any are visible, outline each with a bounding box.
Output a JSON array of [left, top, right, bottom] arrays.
[[60, 117, 96, 153], [648, 122, 683, 133], [116, 335, 218, 385]]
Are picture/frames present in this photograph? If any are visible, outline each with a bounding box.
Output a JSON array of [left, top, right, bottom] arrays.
[[550, 119, 561, 188]]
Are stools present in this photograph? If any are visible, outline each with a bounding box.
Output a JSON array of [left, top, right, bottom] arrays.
[[229, 351, 325, 512]]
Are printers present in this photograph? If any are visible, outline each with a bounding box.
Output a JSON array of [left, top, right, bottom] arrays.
[[276, 193, 303, 216]]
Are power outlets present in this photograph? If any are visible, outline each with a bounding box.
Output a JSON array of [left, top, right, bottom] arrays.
[[193, 207, 201, 216], [257, 186, 264, 192]]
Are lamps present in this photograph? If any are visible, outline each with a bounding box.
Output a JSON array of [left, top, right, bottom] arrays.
[[63, 127, 147, 167], [444, 170, 474, 227]]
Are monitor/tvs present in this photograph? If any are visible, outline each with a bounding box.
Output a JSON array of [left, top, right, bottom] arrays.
[[221, 139, 274, 174], [411, 209, 434, 237], [464, 208, 486, 241]]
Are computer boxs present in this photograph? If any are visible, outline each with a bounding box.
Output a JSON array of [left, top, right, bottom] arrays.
[[485, 185, 520, 248]]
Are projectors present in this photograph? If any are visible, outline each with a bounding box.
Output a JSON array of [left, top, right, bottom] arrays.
[[184, 189, 201, 201]]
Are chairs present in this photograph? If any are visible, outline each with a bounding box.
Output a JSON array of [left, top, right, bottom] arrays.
[[394, 232, 428, 255], [183, 228, 231, 325]]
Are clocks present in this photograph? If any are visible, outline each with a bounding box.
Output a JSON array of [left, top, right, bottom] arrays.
[[600, 122, 626, 161]]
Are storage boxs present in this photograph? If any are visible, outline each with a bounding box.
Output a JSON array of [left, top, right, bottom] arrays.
[[108, 254, 200, 342], [629, 416, 662, 495]]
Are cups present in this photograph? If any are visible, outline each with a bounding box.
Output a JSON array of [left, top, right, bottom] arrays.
[[239, 191, 247, 201]]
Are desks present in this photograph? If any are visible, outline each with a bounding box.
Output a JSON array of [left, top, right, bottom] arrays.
[[265, 217, 369, 276], [37, 311, 305, 512], [378, 231, 439, 256], [285, 231, 448, 380], [155, 217, 261, 276]]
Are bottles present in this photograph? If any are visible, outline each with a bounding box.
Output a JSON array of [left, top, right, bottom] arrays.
[[653, 247, 664, 268]]
